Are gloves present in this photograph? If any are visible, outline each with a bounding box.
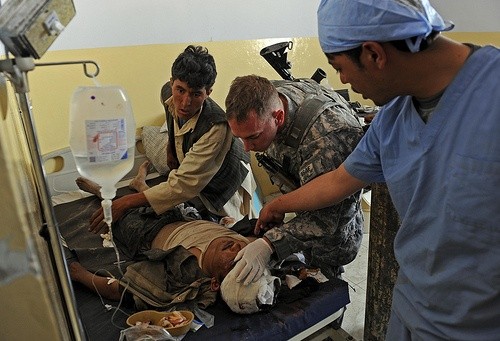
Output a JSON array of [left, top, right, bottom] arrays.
[[232, 238, 273, 286]]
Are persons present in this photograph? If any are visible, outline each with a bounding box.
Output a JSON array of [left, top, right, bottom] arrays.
[[254, 0, 500, 341], [89, 45, 256, 237], [224, 75, 366, 285], [69, 160, 275, 314]]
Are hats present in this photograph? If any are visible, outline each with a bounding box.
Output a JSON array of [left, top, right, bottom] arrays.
[[318, 0, 455, 53]]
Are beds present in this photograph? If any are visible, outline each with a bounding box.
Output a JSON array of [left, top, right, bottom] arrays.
[[42, 144, 354, 341]]
